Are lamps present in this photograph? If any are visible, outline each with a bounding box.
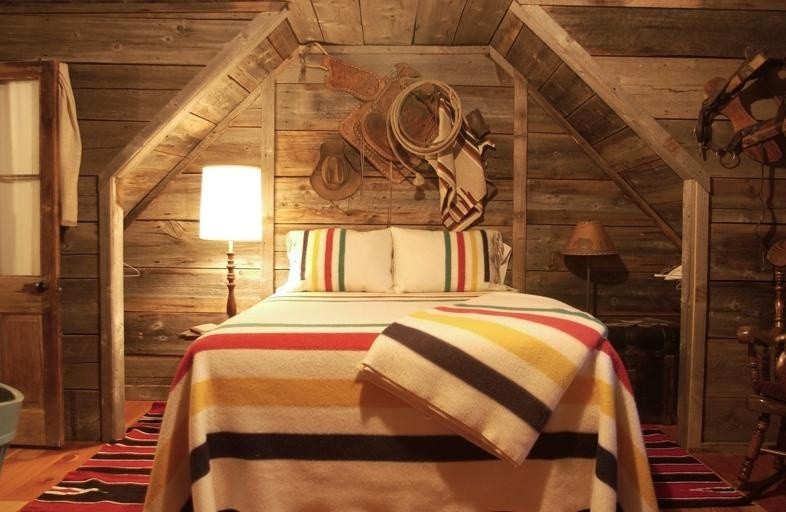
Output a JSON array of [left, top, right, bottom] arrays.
[[197, 163, 270, 318], [559, 220, 620, 314]]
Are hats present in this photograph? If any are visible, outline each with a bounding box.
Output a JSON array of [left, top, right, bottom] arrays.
[[309, 140, 362, 201]]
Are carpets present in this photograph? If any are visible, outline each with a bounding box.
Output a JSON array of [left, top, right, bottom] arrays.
[[15, 404, 768, 512]]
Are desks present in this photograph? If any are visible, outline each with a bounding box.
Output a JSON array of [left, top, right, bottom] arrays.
[[598, 316, 680, 425]]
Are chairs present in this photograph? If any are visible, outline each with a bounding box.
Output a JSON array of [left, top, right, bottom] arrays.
[[734, 238, 785, 490]]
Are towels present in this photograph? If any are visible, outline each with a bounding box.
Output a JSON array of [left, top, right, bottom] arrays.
[[58, 62, 86, 227]]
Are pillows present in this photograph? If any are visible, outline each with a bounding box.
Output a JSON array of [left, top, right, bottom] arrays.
[[282, 224, 510, 296]]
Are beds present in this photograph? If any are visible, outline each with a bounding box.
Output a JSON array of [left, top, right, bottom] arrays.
[[180, 286, 612, 512]]
